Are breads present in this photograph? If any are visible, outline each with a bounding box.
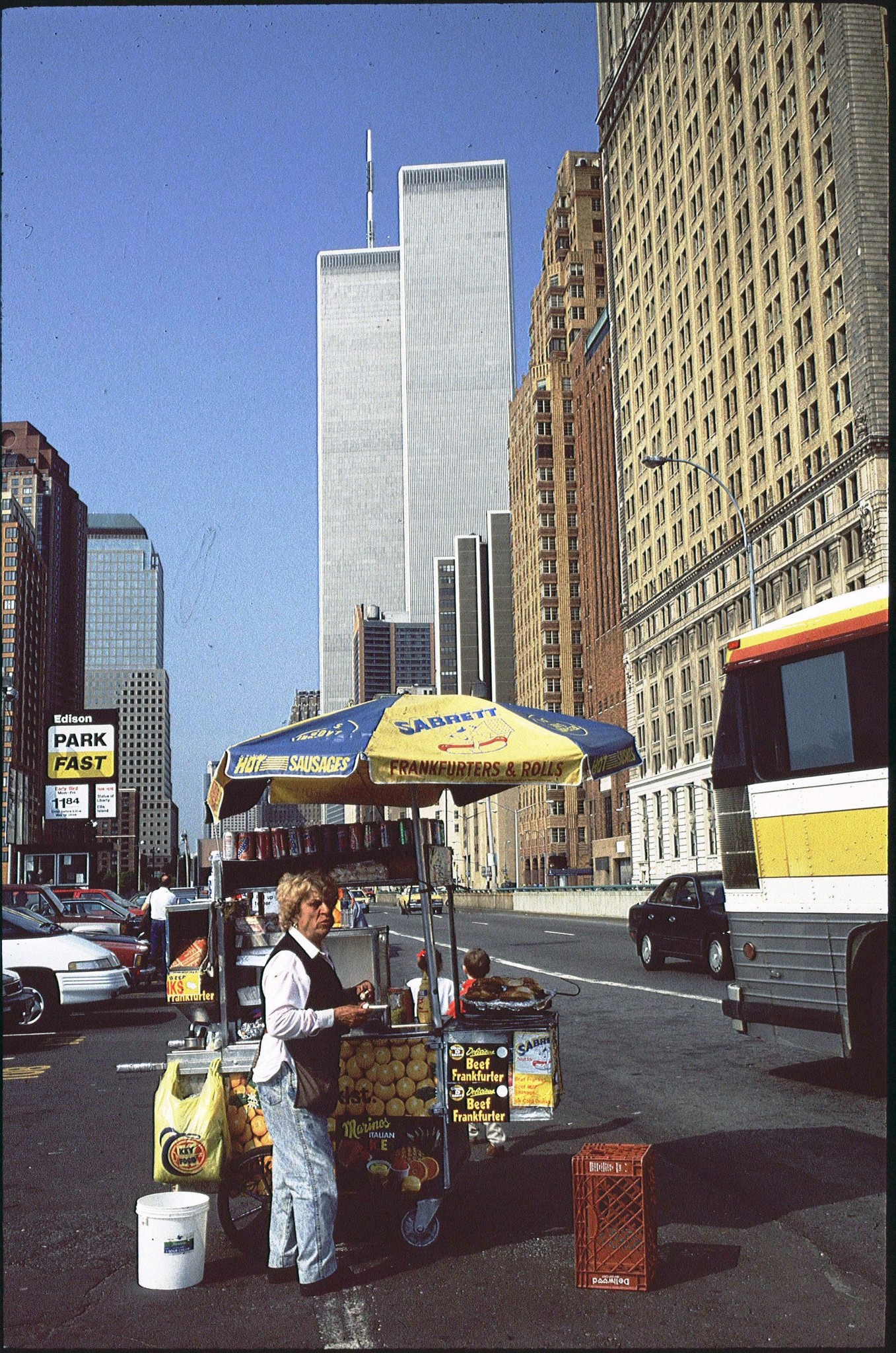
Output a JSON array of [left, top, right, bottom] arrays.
[[464, 975, 544, 1002], [327, 860, 389, 883]]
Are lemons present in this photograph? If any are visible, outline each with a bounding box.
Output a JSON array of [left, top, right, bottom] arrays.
[[401, 1176, 421, 1194]]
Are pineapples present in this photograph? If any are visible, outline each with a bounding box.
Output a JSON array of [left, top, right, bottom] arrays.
[[396, 1126, 442, 1161]]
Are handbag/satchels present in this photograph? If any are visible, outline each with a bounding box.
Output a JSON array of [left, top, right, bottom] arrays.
[[153, 1058, 231, 1183], [140, 892, 151, 933]]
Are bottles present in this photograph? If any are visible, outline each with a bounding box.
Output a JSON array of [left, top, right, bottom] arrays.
[[416, 971, 433, 1024], [340, 889, 353, 929], [401, 979, 414, 1023]]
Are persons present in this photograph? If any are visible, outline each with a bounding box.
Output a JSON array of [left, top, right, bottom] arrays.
[[219, 892, 252, 918], [406, 948, 454, 1018], [446, 948, 504, 1018], [141, 875, 177, 964], [521, 881, 544, 894], [250, 872, 376, 1300], [662, 889, 697, 908], [13, 892, 29, 907], [341, 886, 404, 910]]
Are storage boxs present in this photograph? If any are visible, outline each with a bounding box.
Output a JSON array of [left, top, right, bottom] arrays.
[[572, 1142, 660, 1291]]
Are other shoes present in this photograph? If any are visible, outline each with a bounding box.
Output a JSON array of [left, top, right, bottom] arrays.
[[299, 1266, 351, 1296], [268, 1263, 299, 1283], [469, 1138, 477, 1143], [486, 1144, 505, 1154]]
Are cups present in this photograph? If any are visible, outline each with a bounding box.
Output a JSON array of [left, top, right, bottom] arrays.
[[367, 1160, 390, 1191], [390, 1162, 410, 1192]]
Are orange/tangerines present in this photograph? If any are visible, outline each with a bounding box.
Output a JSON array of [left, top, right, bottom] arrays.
[[221, 1073, 275, 1198], [407, 1157, 440, 1182], [334, 1040, 438, 1118]]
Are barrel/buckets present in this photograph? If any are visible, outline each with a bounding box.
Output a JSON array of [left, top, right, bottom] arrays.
[[135, 1191, 209, 1291]]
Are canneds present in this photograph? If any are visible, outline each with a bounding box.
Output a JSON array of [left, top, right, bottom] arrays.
[[223, 817, 445, 860]]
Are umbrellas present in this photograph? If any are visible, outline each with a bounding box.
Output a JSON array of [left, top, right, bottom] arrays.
[[205, 688, 642, 1029]]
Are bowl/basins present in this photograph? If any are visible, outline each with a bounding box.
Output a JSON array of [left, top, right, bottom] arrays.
[[183, 1036, 205, 1050]]
